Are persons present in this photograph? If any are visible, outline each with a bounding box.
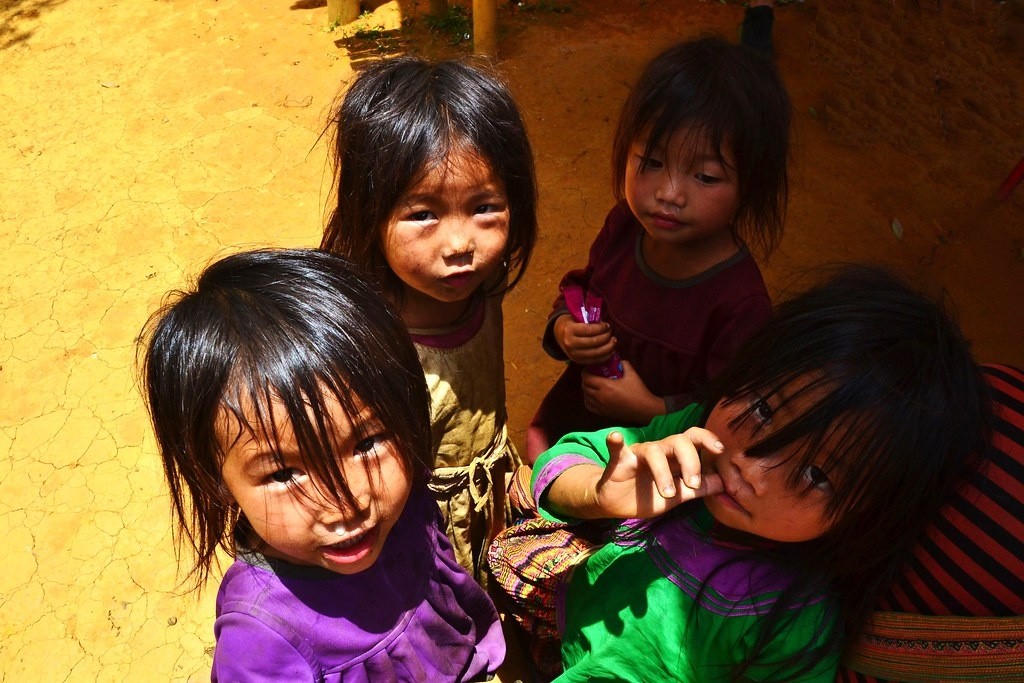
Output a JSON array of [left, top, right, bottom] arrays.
[[523, 33, 793, 468], [319, 57, 539, 633], [531, 267, 992, 683], [145, 243, 530, 683], [740, 0, 776, 66]]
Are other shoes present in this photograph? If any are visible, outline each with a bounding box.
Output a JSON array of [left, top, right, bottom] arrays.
[[737, 4, 774, 50]]
